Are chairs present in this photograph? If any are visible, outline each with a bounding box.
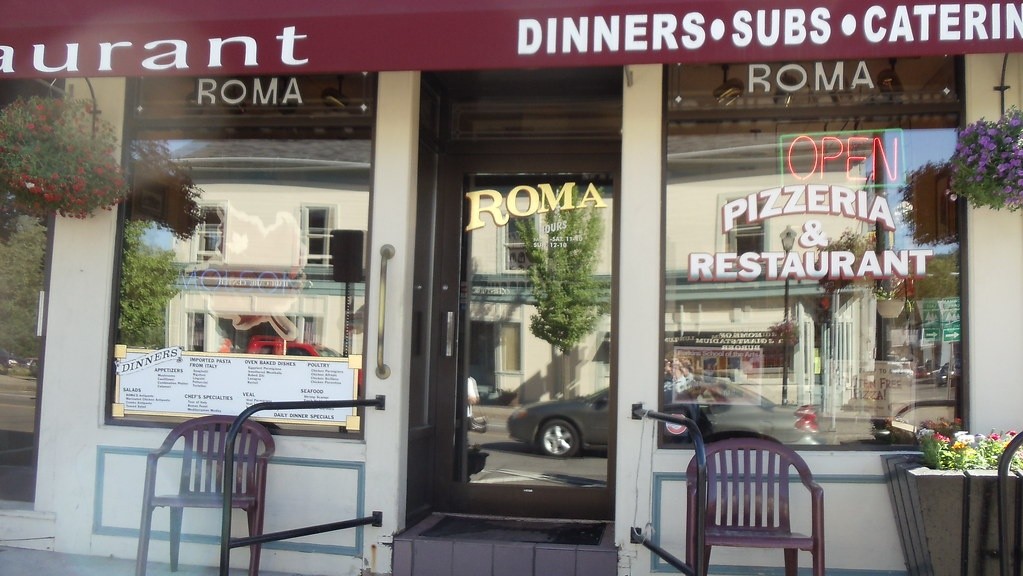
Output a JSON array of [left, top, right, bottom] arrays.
[[135, 414, 275, 576], [685, 437, 825, 576]]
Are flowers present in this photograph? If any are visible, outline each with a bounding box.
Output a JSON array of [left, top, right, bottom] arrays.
[[874, 275, 915, 312], [943, 103, 1023, 212], [914, 426, 1023, 471]]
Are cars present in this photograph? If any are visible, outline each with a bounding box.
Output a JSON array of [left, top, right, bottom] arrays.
[[936, 362, 960, 387], [507, 374, 821, 459]]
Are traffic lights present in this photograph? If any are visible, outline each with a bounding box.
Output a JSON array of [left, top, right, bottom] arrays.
[[816, 278, 832, 323]]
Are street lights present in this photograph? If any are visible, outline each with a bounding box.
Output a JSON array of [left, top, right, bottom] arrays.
[[780, 226, 797, 406]]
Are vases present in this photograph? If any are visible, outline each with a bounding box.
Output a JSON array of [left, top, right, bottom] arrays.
[[876, 300, 906, 318]]
